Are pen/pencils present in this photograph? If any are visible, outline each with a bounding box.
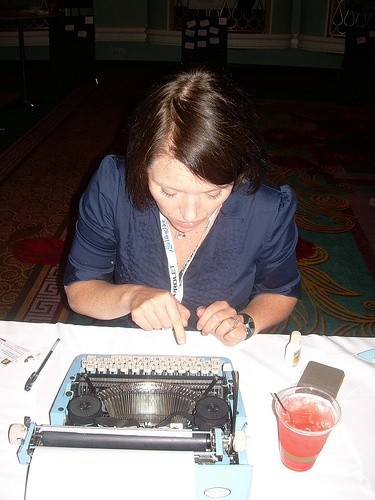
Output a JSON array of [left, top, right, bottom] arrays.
[[24, 338, 60, 392]]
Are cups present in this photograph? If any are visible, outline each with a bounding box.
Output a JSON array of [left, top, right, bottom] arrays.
[[275, 386, 341, 472]]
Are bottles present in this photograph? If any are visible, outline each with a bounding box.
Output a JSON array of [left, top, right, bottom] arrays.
[[285, 331, 302, 367]]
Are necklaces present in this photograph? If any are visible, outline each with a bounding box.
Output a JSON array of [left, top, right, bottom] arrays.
[[156, 204, 187, 240]]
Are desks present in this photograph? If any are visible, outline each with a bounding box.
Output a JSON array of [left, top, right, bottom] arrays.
[[1, 320, 375, 498]]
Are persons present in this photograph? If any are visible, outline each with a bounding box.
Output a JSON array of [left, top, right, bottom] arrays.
[[64, 69, 302, 346]]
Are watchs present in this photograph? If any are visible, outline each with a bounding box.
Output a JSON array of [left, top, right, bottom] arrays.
[[237, 313, 255, 340]]
